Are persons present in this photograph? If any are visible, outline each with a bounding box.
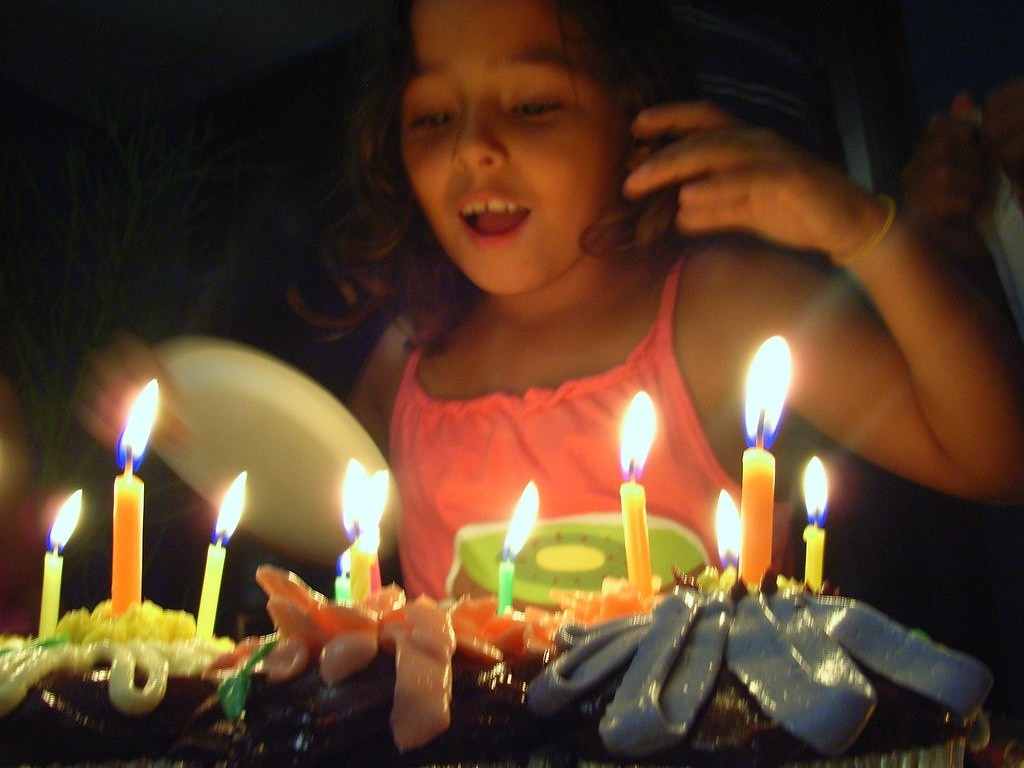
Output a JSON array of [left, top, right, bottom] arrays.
[[72, 2, 1024, 651], [897, 74, 1024, 383]]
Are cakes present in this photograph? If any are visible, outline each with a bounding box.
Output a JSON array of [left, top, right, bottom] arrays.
[[0, 566, 989, 768]]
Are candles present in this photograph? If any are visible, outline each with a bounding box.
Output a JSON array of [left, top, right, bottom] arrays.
[[39, 487, 86, 642], [336, 459, 390, 606], [195, 469, 249, 639], [497, 479, 539, 619], [803, 453, 828, 592], [618, 390, 658, 606], [716, 489, 740, 595], [111, 378, 162, 618], [741, 335, 794, 587]]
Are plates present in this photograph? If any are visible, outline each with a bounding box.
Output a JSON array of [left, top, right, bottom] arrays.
[[147, 337, 399, 569]]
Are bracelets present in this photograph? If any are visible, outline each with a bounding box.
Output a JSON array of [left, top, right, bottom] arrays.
[[828, 191, 895, 267]]
